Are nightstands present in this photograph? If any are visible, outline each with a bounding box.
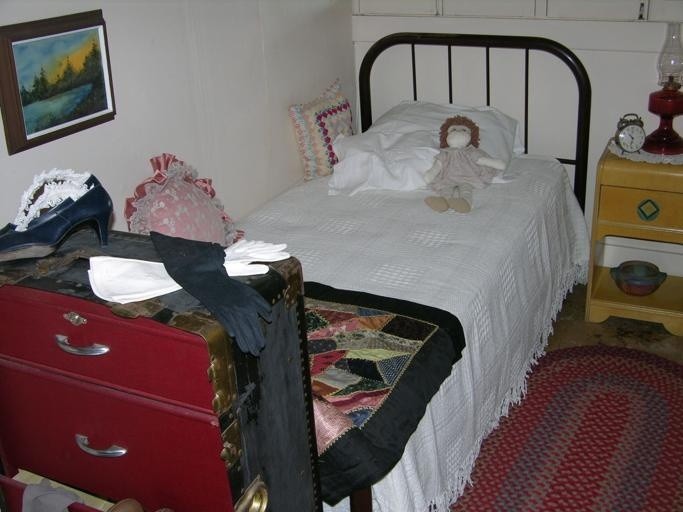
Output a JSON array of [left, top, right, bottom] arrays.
[[0, 223, 323, 512], [584, 135, 683, 338]]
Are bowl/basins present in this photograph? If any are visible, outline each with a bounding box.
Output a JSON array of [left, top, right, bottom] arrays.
[[611, 268, 667, 297]]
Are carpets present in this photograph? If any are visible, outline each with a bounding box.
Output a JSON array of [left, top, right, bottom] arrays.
[[449, 344, 683, 512]]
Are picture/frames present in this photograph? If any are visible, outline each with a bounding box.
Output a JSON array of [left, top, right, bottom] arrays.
[[0, 7, 116, 157]]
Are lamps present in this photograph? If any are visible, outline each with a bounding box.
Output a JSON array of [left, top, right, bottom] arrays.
[[642, 21, 683, 157]]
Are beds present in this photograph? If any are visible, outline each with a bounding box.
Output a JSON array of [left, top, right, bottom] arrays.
[[229, 29, 591, 512]]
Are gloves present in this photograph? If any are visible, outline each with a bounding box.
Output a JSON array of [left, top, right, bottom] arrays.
[[87, 238, 291, 304], [149, 226, 274, 359]]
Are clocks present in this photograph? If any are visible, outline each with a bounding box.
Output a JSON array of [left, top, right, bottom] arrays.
[[615, 113, 645, 156]]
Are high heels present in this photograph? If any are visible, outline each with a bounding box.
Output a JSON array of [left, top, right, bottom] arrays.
[[0, 167, 101, 239], [0, 183, 117, 262]]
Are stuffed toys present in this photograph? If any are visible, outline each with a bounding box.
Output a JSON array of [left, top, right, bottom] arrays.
[[423, 114, 507, 214]]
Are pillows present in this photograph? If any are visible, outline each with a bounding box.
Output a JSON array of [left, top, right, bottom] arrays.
[[289, 76, 356, 184], [367, 100, 516, 185], [126, 151, 243, 251]]
